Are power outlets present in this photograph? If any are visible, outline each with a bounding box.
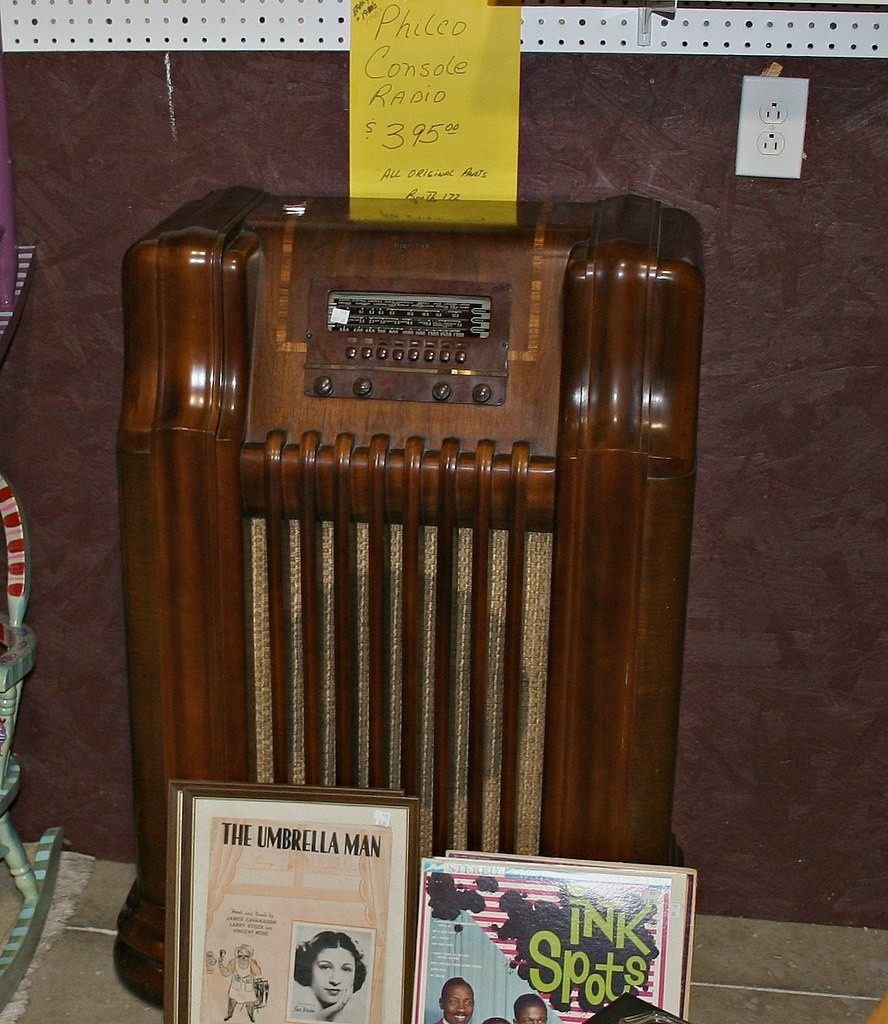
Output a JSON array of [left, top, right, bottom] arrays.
[[736, 70, 809, 176]]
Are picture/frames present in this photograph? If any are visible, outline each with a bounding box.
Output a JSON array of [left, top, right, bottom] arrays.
[[163, 778, 427, 1023]]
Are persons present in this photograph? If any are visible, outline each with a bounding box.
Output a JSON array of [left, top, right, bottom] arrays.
[[513, 993, 548, 1024], [433, 977, 475, 1024], [294, 931, 367, 1022]]
